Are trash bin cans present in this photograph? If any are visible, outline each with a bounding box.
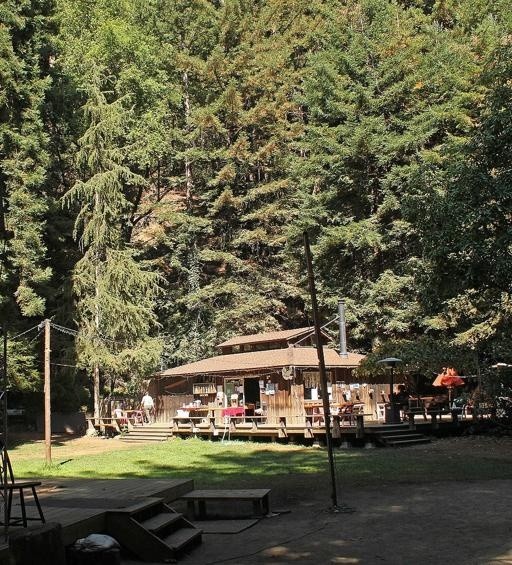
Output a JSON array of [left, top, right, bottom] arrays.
[[385, 403, 400, 424]]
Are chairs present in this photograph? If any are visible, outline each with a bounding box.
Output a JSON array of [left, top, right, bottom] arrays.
[[0, 434, 46, 542]]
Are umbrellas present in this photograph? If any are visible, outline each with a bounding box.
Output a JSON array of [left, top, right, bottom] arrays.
[[431, 367, 466, 409]]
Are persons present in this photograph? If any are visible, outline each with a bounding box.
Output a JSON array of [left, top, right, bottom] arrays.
[[140, 391, 155, 425], [396, 385, 409, 421], [112, 403, 132, 428]]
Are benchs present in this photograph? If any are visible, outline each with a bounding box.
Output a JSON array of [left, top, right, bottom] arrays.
[[303, 413, 372, 427], [228, 415, 266, 431], [179, 487, 273, 522], [171, 416, 216, 430]]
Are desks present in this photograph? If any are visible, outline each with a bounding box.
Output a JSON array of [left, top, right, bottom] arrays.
[[123, 409, 145, 426]]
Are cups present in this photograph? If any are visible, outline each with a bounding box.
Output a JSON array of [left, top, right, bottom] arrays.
[[310, 389, 318, 400], [183, 402, 200, 408]]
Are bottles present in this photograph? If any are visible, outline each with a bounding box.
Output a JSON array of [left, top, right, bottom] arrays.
[[338, 407, 364, 414], [202, 398, 222, 409], [195, 384, 215, 393]]
